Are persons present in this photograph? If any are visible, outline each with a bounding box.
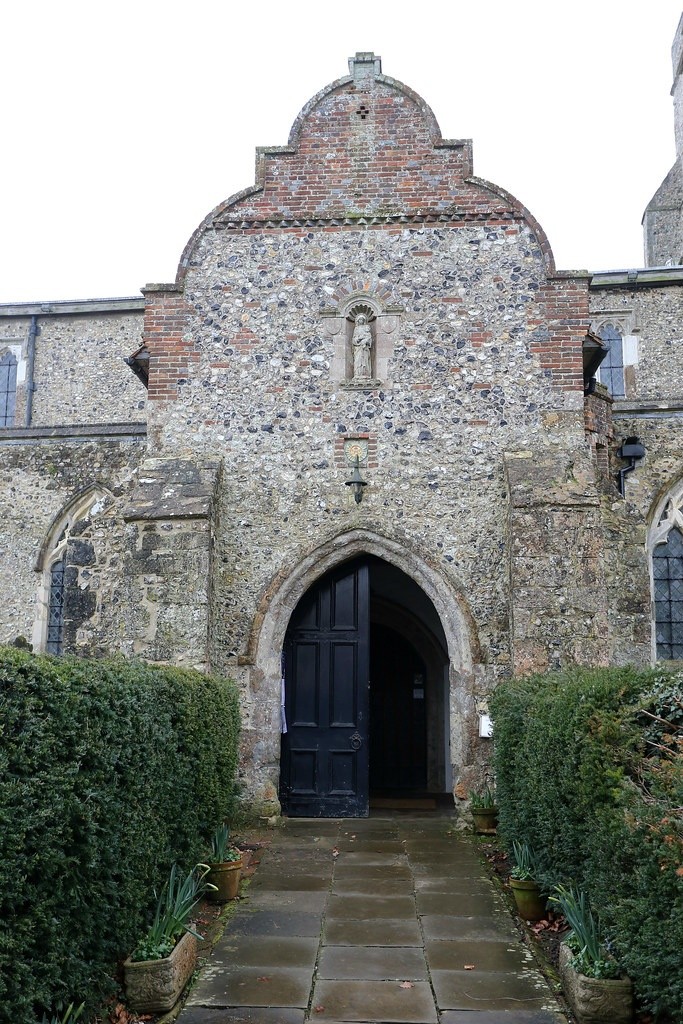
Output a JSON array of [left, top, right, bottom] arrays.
[[352, 317, 372, 379]]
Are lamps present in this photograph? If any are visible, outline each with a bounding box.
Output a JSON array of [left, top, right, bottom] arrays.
[[346, 454, 367, 503]]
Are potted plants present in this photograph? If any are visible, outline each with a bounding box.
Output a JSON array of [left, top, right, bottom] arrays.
[[548, 882, 632, 1024], [508, 838, 550, 921], [123, 863, 219, 1012], [468, 788, 500, 829], [201, 822, 244, 901]]
